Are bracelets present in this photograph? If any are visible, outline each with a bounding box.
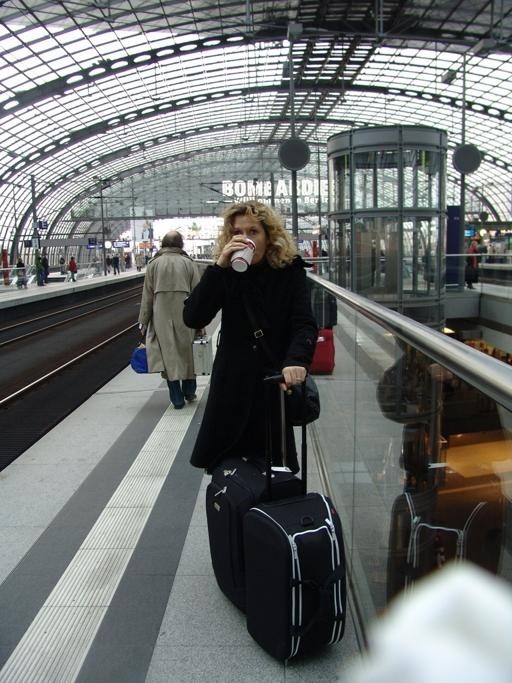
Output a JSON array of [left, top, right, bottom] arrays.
[[138, 322, 145, 329]]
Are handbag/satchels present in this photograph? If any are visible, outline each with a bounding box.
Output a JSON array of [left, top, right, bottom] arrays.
[[310, 288, 338, 329], [284, 374, 320, 427], [130, 329, 148, 373]]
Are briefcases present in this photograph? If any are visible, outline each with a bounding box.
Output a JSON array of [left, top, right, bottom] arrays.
[[193, 334, 213, 376]]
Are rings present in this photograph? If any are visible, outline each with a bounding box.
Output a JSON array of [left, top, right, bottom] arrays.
[[295, 377, 303, 382]]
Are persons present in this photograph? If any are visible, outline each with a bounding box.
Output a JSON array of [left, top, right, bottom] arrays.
[[16, 256, 29, 290], [392, 336, 502, 447], [67, 256, 78, 282], [136, 229, 207, 408], [59, 256, 67, 275], [41, 254, 50, 283], [107, 253, 131, 274], [184, 199, 319, 481], [465, 240, 481, 289], [311, 281, 338, 330]]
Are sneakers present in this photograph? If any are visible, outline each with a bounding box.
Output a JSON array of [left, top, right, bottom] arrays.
[[174, 394, 197, 409]]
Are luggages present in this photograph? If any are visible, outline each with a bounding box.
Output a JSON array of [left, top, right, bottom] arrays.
[[205, 373, 346, 660], [309, 330, 336, 375], [377, 349, 505, 605]]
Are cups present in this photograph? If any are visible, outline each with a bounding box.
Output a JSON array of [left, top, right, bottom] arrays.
[[230, 238, 257, 272]]
